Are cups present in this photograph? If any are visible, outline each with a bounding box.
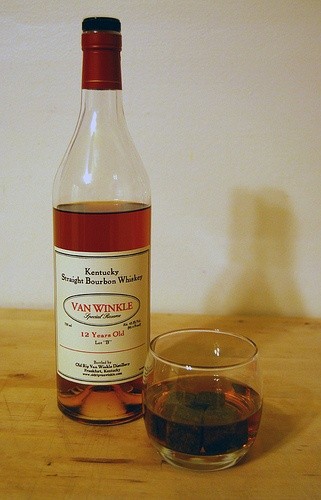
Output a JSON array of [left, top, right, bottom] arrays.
[[142, 328, 263, 471]]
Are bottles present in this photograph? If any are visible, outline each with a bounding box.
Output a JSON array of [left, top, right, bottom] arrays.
[[52, 16, 152, 426]]
